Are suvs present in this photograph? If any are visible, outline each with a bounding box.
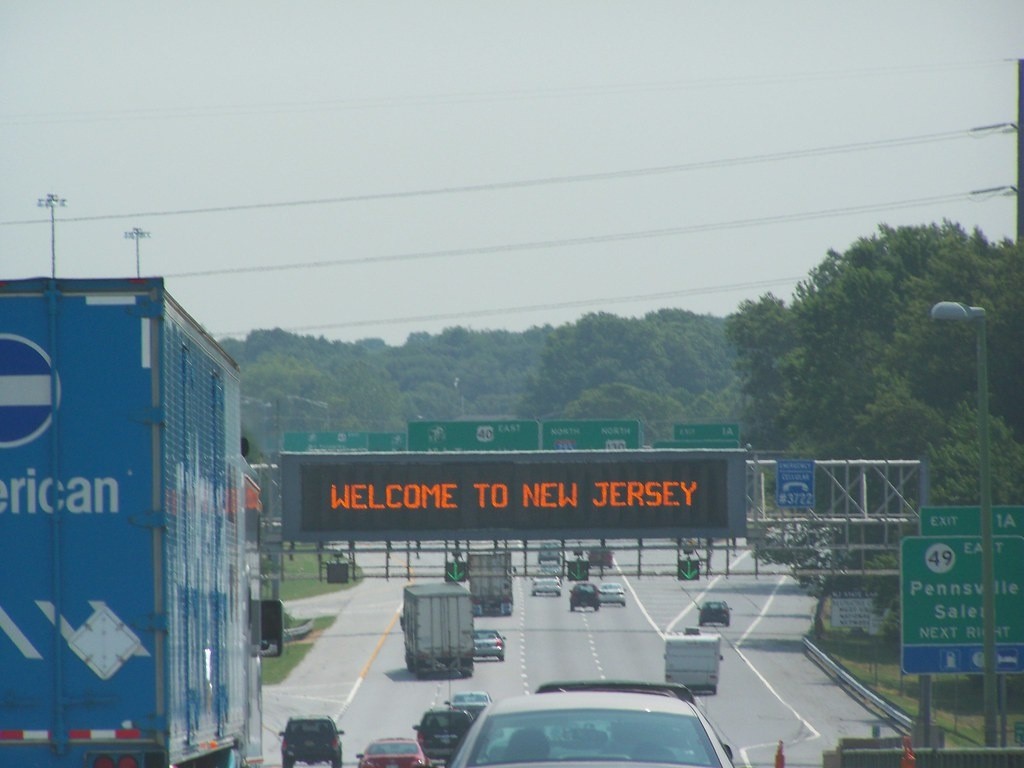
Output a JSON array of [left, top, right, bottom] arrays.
[[538, 542, 562, 564], [411, 708, 477, 760], [697, 602, 733, 627], [568, 582, 600, 612], [586, 545, 615, 569], [278, 715, 344, 768]]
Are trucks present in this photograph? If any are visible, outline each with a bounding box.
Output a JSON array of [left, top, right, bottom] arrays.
[[468, 553, 516, 612], [400, 583, 475, 677], [0, 276, 287, 767]]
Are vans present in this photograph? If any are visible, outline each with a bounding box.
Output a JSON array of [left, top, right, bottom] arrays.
[[663, 628, 723, 695]]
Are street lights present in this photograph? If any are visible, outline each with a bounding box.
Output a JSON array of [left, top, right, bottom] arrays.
[[125, 227, 152, 278], [35, 193, 65, 279], [931, 298, 1002, 751]]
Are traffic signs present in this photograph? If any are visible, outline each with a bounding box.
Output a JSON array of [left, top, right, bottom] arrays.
[[540, 420, 645, 452], [405, 418, 542, 453], [900, 535, 1022, 677]]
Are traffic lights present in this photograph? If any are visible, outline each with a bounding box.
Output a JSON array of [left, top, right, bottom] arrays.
[[445, 562, 467, 581], [569, 560, 590, 581], [677, 560, 698, 580]]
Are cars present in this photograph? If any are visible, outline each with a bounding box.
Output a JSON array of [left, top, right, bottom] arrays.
[[445, 691, 734, 767], [355, 737, 429, 767], [445, 692, 494, 720], [598, 584, 626, 607], [530, 573, 562, 597], [473, 629, 506, 662]]
